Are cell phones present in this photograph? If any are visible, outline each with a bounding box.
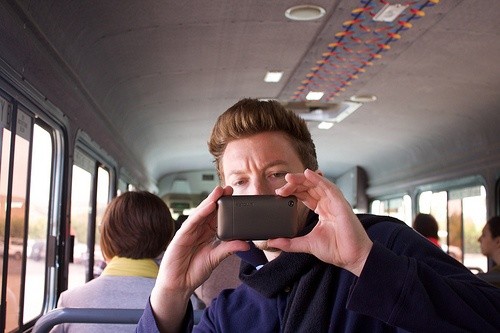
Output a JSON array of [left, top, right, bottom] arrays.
[[216, 195, 298, 241]]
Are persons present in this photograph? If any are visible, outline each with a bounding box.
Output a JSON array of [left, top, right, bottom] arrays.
[[136, 99, 500, 333], [413, 213, 441, 249], [475, 218, 500, 288], [49, 192, 206, 333]]
